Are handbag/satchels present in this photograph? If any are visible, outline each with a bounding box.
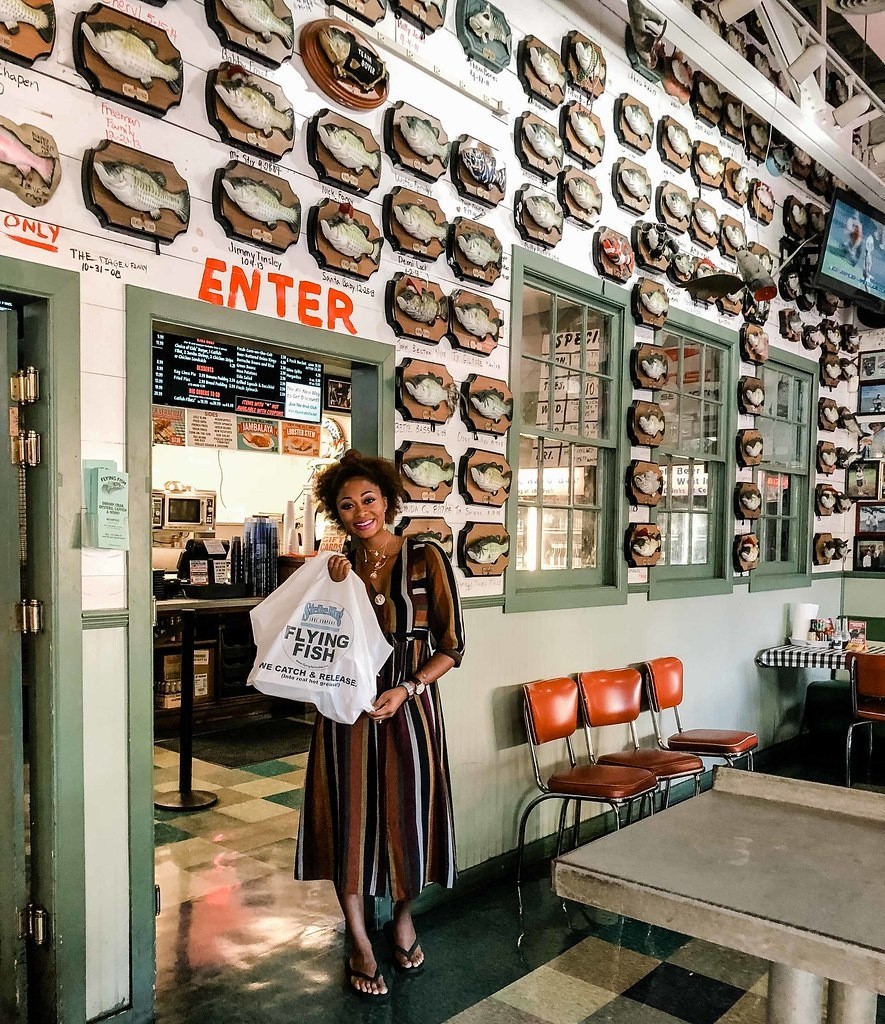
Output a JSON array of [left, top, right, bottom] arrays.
[[245, 551, 394, 725]]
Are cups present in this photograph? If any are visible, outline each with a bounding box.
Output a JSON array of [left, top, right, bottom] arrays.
[[232, 517, 279, 597], [283, 495, 315, 555]]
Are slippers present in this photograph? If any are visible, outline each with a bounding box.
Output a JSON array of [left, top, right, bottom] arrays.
[[383, 920, 426, 974], [345, 956, 390, 1002]]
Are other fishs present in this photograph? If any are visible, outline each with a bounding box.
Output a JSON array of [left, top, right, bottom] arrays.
[[79, 21, 184, 97], [215, 78, 294, 144], [90, 158, 191, 225], [0, 0, 56, 45], [218, 175, 302, 237], [404, 371, 457, 412], [319, 214, 384, 265], [0, 124, 58, 187], [453, 3, 872, 565], [361, 0, 388, 10], [411, 530, 454, 560], [317, 25, 390, 96], [401, 455, 455, 491], [414, 0, 448, 19], [392, 203, 449, 248], [219, 0, 296, 52], [397, 115, 451, 172], [317, 123, 382, 179], [396, 287, 449, 327]]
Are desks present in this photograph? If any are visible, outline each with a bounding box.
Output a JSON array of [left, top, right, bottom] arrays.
[[150, 597, 265, 809], [551, 765, 885, 1024], [755, 640, 885, 744]]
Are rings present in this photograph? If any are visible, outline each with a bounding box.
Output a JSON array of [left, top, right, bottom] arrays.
[[379, 719, 383, 724], [334, 564, 340, 569]]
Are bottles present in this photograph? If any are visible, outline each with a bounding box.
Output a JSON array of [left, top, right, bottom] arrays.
[[809, 616, 850, 649]]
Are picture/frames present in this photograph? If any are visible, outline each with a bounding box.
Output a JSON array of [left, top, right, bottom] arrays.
[[855, 502, 885, 536], [857, 349, 885, 417], [845, 460, 885, 502], [853, 536, 885, 572]]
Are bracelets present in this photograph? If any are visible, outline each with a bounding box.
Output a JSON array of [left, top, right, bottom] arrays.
[[399, 681, 414, 703]]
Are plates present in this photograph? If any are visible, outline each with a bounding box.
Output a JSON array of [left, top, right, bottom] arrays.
[[285, 436, 315, 454], [243, 433, 274, 450]]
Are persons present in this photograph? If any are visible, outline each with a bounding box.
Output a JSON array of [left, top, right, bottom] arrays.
[[293, 448, 466, 1001], [855, 354, 885, 568]]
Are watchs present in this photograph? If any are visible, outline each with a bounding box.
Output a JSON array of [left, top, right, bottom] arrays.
[[409, 675, 426, 696]]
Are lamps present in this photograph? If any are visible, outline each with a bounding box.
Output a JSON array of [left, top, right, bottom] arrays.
[[871, 141, 885, 164], [786, 24, 828, 84], [711, 0, 761, 26], [830, 73, 870, 129]]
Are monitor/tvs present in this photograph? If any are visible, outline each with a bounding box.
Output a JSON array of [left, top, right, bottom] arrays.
[[812, 186, 885, 314]]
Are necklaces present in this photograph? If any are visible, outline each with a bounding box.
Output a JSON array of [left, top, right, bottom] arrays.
[[358, 533, 398, 579]]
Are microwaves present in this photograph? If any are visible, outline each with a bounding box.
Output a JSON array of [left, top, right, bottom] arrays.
[[152, 492, 213, 528]]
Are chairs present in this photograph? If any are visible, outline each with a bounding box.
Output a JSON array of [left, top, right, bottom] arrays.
[[573, 668, 707, 855], [844, 652, 885, 789], [516, 677, 659, 882], [642, 657, 758, 812], [795, 615, 885, 744]]
